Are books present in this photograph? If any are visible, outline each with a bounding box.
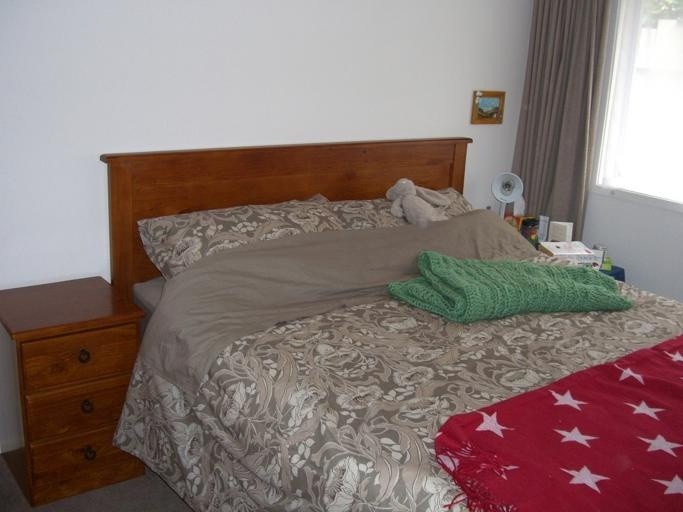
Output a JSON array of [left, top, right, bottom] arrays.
[[537, 239, 593, 260]]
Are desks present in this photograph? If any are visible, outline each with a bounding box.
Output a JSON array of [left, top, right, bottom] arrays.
[[0, 277, 146, 506]]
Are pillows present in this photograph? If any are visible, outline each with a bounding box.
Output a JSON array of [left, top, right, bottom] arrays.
[[319, 188, 477, 229], [136, 198, 356, 289]]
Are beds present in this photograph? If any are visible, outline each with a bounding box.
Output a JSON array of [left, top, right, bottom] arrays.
[[98, 136, 683, 511]]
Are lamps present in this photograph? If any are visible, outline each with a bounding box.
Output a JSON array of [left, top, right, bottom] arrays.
[[491, 171, 526, 216]]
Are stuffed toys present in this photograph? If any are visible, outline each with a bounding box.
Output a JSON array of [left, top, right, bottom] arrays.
[[385, 178, 450, 224]]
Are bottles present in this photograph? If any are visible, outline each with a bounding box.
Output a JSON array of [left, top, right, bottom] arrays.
[[520, 219, 540, 251]]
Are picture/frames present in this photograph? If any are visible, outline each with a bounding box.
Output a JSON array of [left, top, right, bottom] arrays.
[[468, 88, 507, 127]]
